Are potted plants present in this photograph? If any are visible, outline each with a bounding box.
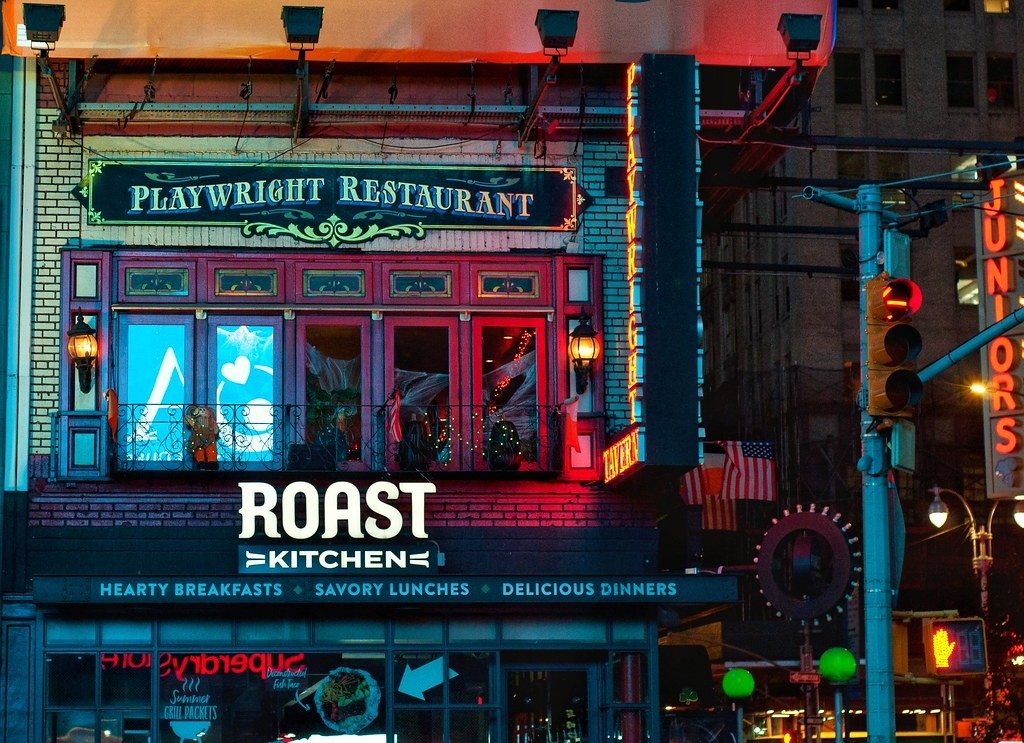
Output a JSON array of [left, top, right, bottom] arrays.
[[305, 373, 359, 461]]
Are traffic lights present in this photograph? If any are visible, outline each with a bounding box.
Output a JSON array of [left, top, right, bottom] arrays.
[[867, 278, 924, 418], [922, 617, 988, 678]]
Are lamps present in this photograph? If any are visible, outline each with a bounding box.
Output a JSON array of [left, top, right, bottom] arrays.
[[777, 13, 823, 59], [535, 9, 580, 55], [568, 306, 601, 395], [22, 3, 66, 57], [975, 152, 1010, 178], [280, 6, 325, 51], [66, 306, 98, 394]]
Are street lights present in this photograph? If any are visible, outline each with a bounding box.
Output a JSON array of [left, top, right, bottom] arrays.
[[721, 668, 755, 743], [927, 484, 1024, 653], [819, 647, 856, 743]]
